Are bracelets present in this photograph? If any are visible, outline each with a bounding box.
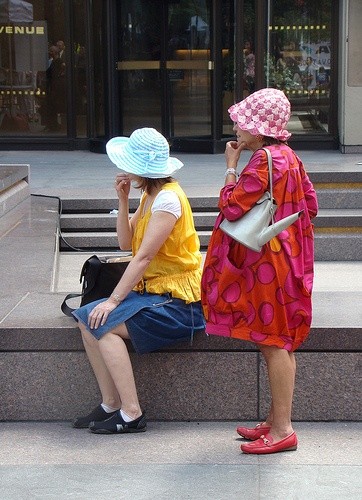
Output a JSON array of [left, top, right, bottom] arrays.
[[109, 292, 123, 302], [223, 167, 239, 177]]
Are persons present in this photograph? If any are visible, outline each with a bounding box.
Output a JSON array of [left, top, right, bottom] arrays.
[[241, 39, 317, 91], [39, 38, 67, 135], [201, 88, 318, 454], [73, 127, 205, 433]]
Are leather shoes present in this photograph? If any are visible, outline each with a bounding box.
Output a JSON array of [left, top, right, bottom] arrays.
[[241, 431, 298, 454], [237, 424, 272, 440]]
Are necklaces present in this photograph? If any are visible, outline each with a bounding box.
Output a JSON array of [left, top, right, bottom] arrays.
[[142, 193, 151, 214]]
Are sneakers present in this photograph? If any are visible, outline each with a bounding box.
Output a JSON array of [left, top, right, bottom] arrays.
[[88, 410, 147, 434], [73, 402, 120, 428]]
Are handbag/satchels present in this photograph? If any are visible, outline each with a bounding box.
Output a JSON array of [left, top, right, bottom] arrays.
[[61, 255, 131, 317], [219, 148, 304, 252]]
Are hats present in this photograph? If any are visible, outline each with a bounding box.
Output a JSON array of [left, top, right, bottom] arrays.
[[106, 128, 184, 179], [228, 88, 292, 141]]
